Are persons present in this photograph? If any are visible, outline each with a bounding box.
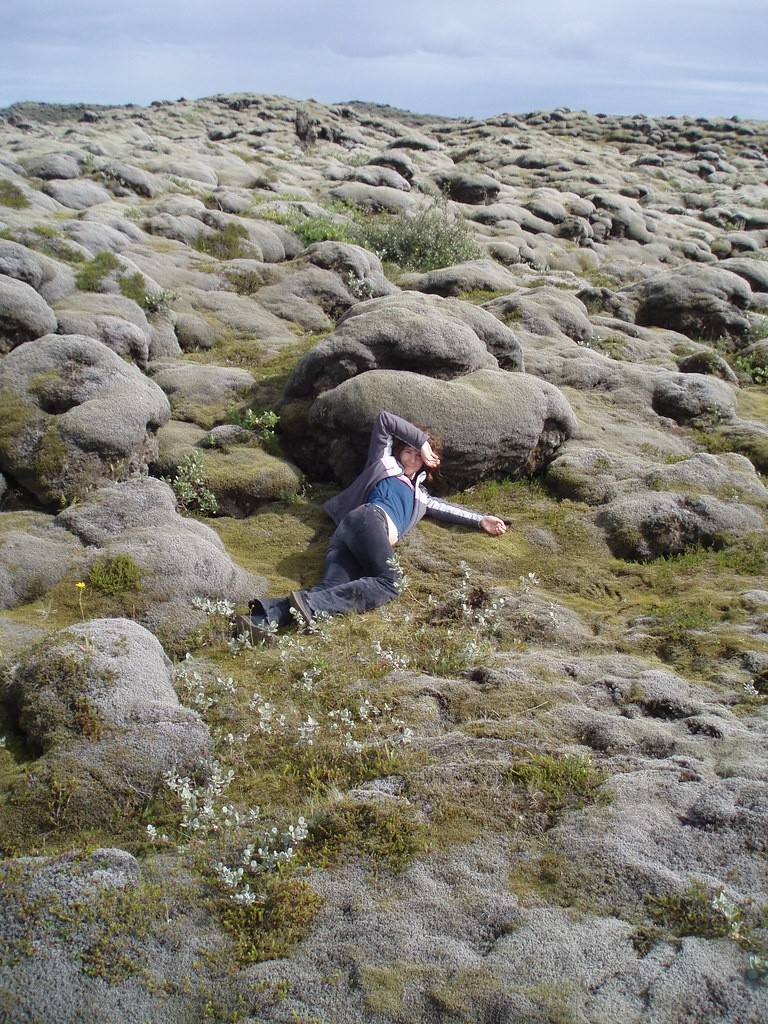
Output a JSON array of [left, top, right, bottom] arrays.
[[238, 411, 506, 639]]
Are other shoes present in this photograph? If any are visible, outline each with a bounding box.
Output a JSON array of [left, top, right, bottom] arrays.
[[290, 591, 316, 624], [238, 613, 276, 646]]
